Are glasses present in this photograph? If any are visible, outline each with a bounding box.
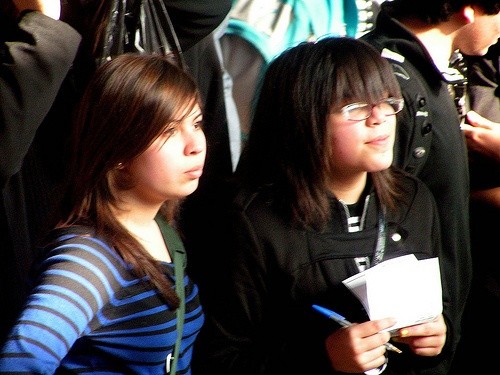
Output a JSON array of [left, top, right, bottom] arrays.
[[327, 96, 404, 120]]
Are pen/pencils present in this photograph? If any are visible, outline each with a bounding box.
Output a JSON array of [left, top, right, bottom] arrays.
[[311, 304, 404, 357]]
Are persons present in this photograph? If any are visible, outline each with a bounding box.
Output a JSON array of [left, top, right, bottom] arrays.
[[200, 36, 462, 375], [0, 54, 206, 375], [215, 0, 359, 175], [0, 0, 233, 344], [356, 0, 500, 375]]
